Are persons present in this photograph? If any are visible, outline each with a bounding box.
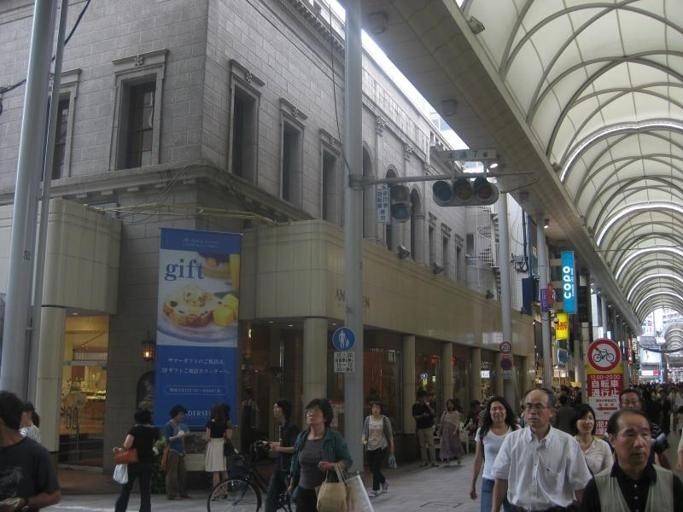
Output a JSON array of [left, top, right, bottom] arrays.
[[261, 401, 300, 512], [241, 388, 256, 407], [115, 411, 155, 512], [287, 399, 353, 512], [163, 406, 193, 500], [222, 403, 234, 491], [18, 402, 40, 443], [0, 391, 62, 512], [204, 406, 229, 501], [361, 399, 395, 497], [412, 382, 683, 512]]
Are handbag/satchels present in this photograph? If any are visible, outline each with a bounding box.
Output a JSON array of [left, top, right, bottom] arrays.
[[223, 421, 234, 456], [336, 462, 374, 512], [112, 426, 139, 464], [315, 463, 351, 512]]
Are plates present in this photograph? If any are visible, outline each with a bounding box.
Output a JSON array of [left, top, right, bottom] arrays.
[[201, 267, 228, 280], [156, 311, 237, 343]]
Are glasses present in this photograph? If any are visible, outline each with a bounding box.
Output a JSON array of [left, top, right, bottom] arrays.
[[522, 404, 552, 411]]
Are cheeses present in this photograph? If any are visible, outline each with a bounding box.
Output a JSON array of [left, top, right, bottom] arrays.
[[222, 294, 239, 320], [212, 303, 234, 325]]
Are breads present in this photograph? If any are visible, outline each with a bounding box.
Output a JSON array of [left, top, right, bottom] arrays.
[[163, 286, 221, 327]]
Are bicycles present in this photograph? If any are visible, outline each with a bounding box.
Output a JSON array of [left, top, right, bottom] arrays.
[[205, 440, 298, 512]]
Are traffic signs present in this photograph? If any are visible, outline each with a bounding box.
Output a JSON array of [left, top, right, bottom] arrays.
[[426, 146, 500, 163]]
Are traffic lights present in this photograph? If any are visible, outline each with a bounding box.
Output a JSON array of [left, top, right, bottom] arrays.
[[428, 174, 501, 207], [389, 184, 410, 222]]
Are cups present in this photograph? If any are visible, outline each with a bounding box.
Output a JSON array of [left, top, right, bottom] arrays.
[[227, 254, 239, 291]]
